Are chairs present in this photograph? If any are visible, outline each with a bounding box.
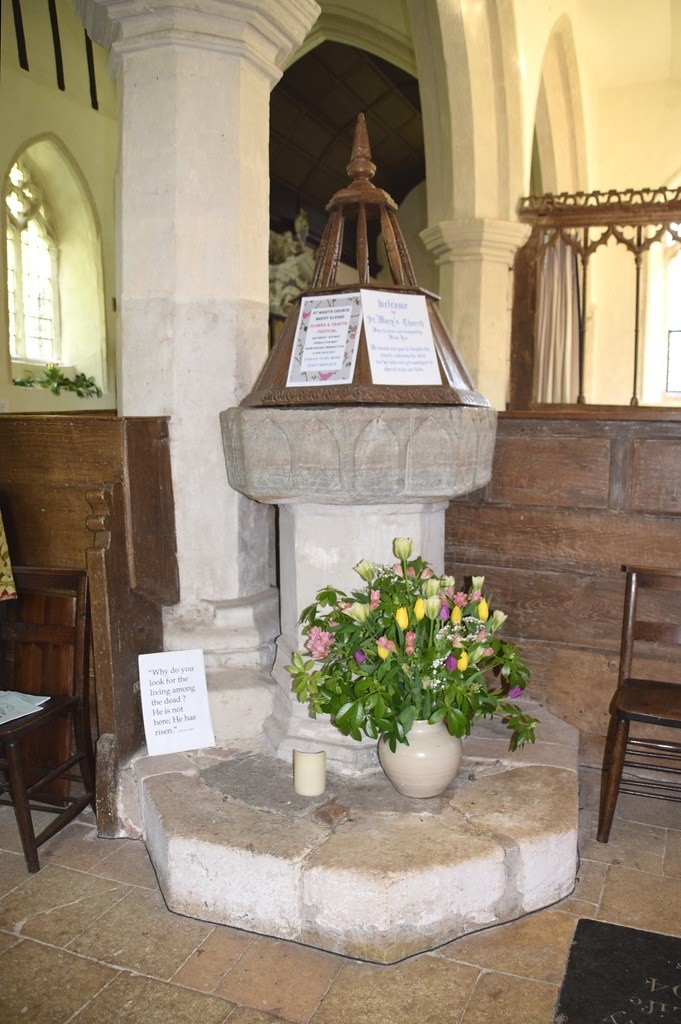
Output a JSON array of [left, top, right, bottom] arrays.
[[597, 563, 681, 842], [0, 568, 97, 873]]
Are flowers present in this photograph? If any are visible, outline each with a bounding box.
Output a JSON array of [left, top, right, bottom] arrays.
[[286, 537, 539, 754]]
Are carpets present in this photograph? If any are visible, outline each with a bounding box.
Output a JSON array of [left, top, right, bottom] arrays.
[[555, 918, 681, 1024]]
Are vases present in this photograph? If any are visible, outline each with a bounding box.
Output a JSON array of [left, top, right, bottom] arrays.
[[379, 717, 463, 798]]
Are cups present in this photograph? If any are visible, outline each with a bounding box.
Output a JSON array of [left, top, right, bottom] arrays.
[[292, 749, 326, 796]]
